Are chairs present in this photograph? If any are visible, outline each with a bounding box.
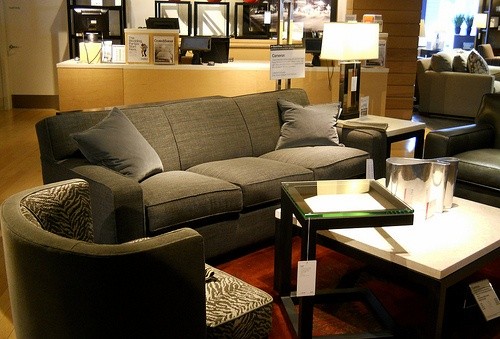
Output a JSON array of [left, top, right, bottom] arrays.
[[423, 93, 500, 207], [478, 44, 500, 65], [1, 178, 274, 339]]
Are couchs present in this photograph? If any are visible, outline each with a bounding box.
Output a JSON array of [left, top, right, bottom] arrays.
[[418, 49, 500, 119], [35, 89, 390, 260]]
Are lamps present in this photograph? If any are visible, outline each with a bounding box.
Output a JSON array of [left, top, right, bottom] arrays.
[[321, 23, 379, 119]]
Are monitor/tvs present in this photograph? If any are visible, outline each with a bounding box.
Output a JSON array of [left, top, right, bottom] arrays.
[[71, 8, 109, 38], [181, 37, 230, 64]]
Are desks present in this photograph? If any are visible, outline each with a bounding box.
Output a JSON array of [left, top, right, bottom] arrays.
[[273, 177, 500, 339], [340, 116, 427, 159]]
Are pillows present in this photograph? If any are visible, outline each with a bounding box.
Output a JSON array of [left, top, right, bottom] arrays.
[[276, 98, 345, 149], [68, 106, 165, 182], [430, 47, 491, 75]]
